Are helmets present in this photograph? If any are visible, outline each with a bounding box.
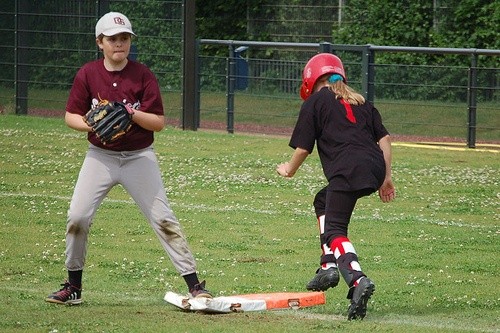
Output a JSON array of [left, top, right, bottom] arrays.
[[300, 53, 346, 101]]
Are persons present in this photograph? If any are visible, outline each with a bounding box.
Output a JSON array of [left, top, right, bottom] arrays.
[[46, 11, 213, 304], [276, 54, 397, 322]]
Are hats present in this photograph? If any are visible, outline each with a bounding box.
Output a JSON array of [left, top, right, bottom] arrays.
[[96, 12, 137, 38]]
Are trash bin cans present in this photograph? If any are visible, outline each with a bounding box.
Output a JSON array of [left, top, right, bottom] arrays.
[[127, 45, 137, 61], [234, 46, 250, 90]]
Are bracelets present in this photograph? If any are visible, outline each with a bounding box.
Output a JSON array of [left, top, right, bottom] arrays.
[[285, 168, 296, 176]]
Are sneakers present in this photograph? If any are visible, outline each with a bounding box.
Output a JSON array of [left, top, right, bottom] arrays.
[[46, 280, 82, 304], [306, 267, 339, 292], [346, 277, 375, 321], [189, 280, 212, 298]]
